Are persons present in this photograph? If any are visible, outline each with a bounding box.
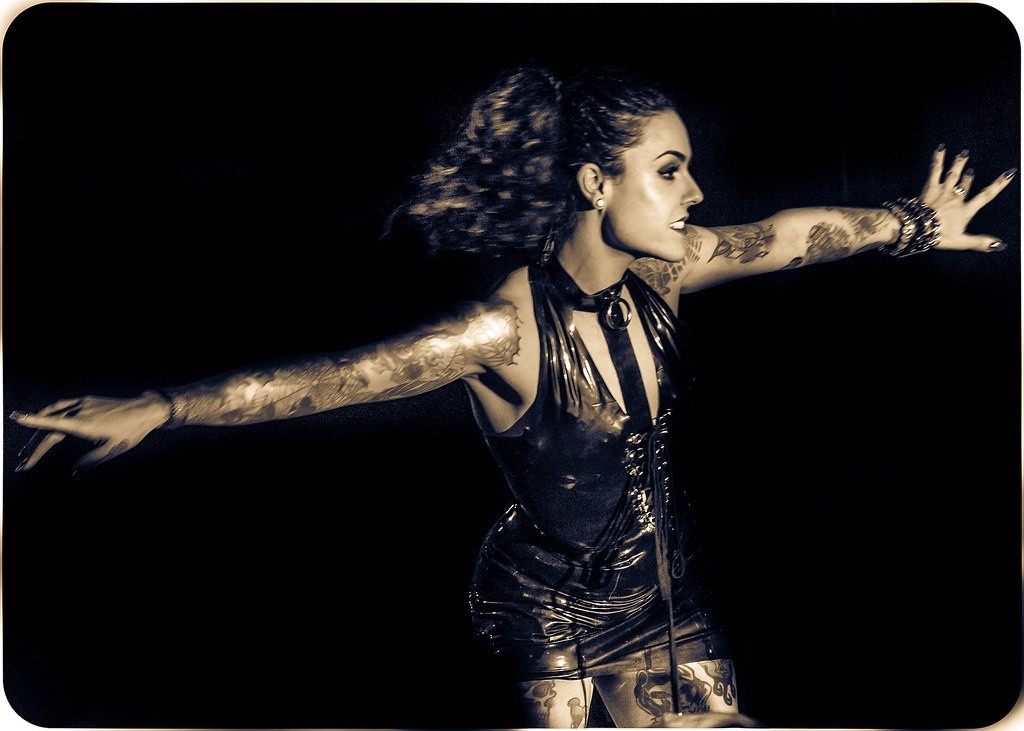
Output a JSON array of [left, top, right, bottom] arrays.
[[6, 53, 1017, 729]]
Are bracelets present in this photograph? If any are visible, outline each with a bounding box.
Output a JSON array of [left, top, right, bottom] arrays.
[[880, 195, 942, 258]]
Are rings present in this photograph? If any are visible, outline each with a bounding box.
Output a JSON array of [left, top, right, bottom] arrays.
[[950, 186, 967, 198]]
